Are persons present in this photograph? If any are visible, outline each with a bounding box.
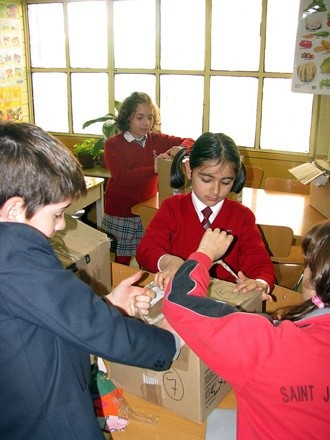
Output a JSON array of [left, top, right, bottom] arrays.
[[0, 122, 184, 440], [135, 131, 276, 303], [101, 92, 197, 266], [161, 218, 330, 440]]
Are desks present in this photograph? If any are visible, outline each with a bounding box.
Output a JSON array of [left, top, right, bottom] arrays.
[[65, 177, 106, 230], [108, 262, 236, 440], [131, 186, 327, 240]]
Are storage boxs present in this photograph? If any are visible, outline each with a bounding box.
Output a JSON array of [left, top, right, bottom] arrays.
[[49, 213, 262, 425], [157, 155, 244, 209], [287, 158, 330, 220]]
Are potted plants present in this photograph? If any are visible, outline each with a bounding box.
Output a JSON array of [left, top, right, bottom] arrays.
[[72, 139, 103, 169]]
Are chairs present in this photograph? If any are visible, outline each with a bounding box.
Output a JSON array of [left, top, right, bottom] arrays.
[[258, 219, 303, 280], [245, 167, 310, 195]]
